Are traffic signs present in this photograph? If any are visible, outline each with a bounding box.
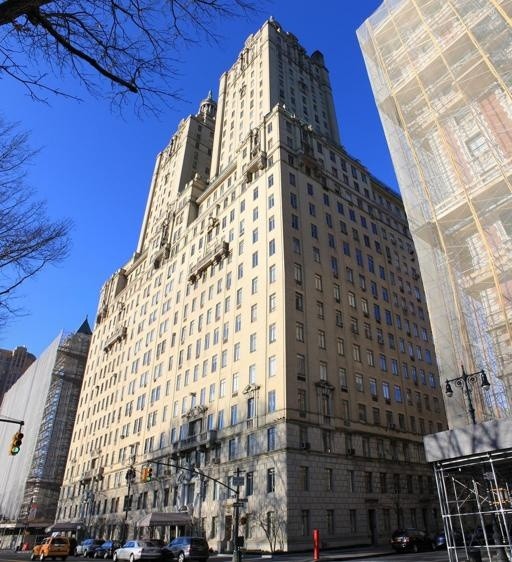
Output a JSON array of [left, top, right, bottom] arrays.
[[232, 503, 245, 509], [237, 499, 249, 503]]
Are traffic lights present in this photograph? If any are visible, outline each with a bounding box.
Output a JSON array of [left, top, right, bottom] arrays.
[[8, 431, 24, 456], [144, 467, 153, 483]]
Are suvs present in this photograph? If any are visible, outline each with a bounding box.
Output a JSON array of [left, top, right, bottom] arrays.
[[29, 537, 70, 562], [390, 527, 436, 553]]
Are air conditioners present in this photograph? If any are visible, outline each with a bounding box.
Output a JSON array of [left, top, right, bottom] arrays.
[[301, 441, 311, 448], [211, 457, 220, 464], [345, 447, 356, 455]]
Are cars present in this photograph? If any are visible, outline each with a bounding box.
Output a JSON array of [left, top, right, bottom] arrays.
[[74, 537, 210, 562], [434, 528, 465, 549], [467, 527, 491, 545]]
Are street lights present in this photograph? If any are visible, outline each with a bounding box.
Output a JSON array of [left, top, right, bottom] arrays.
[[121, 465, 136, 543], [442, 365, 510, 562]]
